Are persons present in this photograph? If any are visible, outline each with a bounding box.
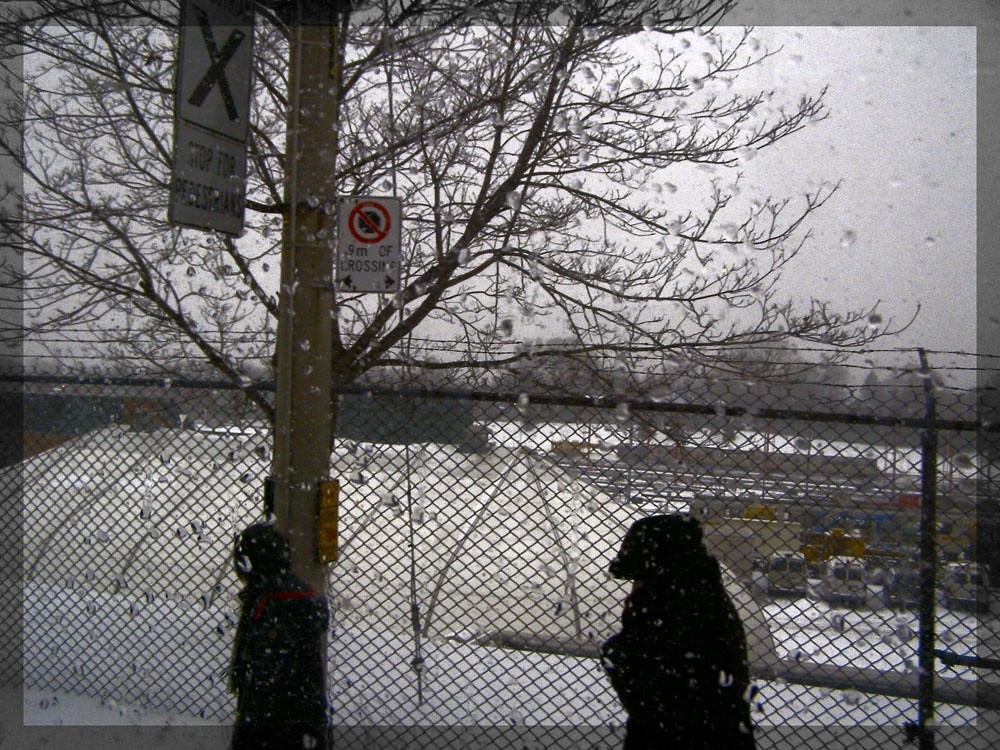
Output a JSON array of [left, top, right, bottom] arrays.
[[226, 525, 335, 750], [596, 512, 758, 750]]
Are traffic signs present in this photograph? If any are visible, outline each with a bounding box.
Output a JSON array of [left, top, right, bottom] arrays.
[[336, 196, 402, 293]]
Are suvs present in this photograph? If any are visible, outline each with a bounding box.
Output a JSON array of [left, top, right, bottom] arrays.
[[763, 551, 810, 597], [941, 562, 991, 612], [821, 555, 868, 604]]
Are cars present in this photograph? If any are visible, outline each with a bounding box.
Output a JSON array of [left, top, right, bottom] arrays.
[[882, 567, 921, 604]]
